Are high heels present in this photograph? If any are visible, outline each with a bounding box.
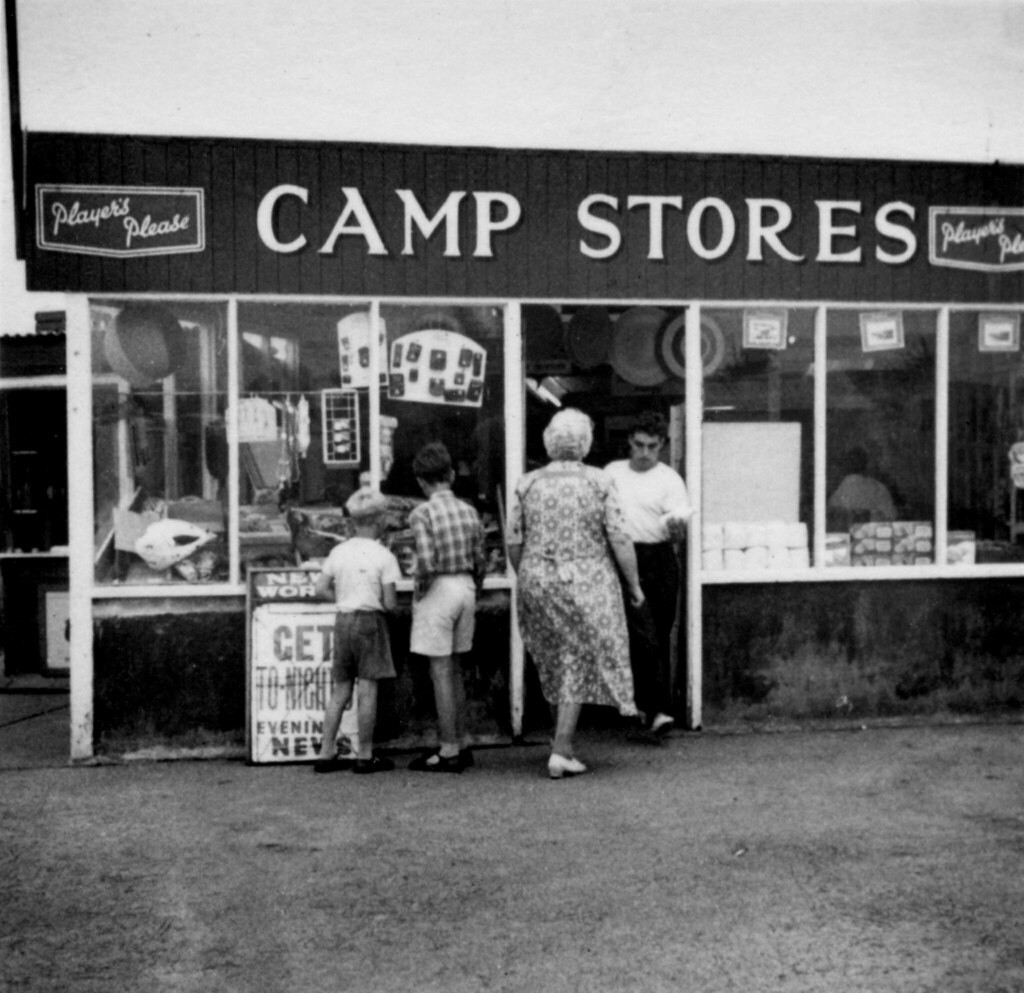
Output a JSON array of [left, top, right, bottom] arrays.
[[546, 732, 556, 749], [548, 754, 587, 778]]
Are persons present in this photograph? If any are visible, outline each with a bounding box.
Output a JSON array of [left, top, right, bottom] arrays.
[[507, 408, 687, 778], [313, 485, 404, 774], [408, 439, 488, 774], [829, 443, 900, 522]]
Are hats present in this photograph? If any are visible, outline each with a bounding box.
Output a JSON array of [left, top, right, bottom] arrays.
[[345, 486, 387, 517]]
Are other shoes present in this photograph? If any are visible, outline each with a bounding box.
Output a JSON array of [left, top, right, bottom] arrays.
[[355, 756, 396, 773], [409, 746, 475, 772], [650, 713, 675, 737], [314, 753, 351, 773]]
